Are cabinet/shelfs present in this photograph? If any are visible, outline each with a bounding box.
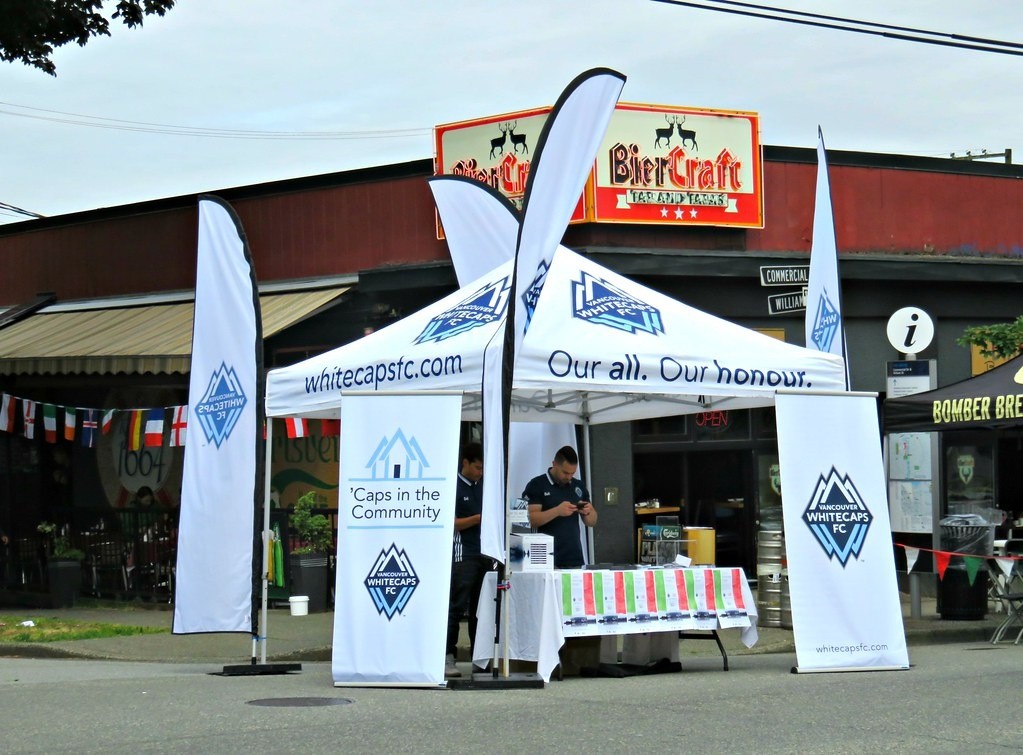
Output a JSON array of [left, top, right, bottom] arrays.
[[638, 528, 716, 566]]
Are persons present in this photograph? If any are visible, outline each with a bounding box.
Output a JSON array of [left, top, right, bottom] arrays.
[[443, 441, 504, 679], [126, 486, 162, 568], [522, 446, 597, 570]]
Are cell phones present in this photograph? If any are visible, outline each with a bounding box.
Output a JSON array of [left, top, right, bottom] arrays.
[[576, 503, 588, 509]]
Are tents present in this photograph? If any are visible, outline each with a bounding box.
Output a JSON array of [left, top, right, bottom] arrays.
[[251, 236, 1023, 679]]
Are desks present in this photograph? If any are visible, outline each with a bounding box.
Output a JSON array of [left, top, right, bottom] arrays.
[[994, 540, 1015, 615], [471, 567, 759, 683]]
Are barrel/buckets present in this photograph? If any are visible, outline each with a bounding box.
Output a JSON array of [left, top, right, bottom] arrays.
[[289, 596, 309, 616], [756, 515, 793, 629]]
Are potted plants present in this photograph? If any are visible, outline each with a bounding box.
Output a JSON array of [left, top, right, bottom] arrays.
[[38, 522, 86, 607], [288, 493, 333, 612]]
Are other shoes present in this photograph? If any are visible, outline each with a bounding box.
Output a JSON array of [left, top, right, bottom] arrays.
[[445, 654, 462, 678]]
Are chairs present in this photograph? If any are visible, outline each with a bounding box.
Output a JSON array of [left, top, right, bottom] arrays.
[[985, 539, 1023, 645]]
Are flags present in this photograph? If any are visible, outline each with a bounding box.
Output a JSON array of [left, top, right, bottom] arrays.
[[0, 391, 192, 450], [285, 418, 310, 438], [321, 419, 340, 437], [260, 419, 270, 441]]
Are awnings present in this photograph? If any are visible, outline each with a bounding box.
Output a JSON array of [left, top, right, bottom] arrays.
[[0, 282, 351, 374]]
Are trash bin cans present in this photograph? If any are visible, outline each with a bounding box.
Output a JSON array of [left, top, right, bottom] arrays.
[[938, 513, 994, 619]]
